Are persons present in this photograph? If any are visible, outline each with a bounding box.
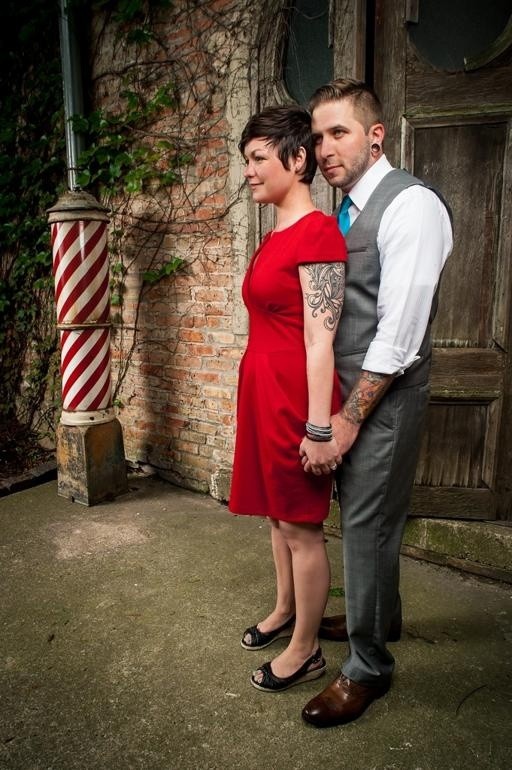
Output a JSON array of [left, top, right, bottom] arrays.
[[227, 104, 350, 694], [300, 78, 454, 730]]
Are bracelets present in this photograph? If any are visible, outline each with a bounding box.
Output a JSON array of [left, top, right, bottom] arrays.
[[304, 421, 335, 441]]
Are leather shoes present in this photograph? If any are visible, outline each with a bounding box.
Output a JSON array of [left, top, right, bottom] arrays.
[[318, 614, 402, 642], [301, 673, 391, 727]]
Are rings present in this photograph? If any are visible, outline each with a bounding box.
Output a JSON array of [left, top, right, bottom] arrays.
[[332, 462, 340, 471]]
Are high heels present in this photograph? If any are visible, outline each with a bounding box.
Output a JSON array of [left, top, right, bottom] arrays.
[[250, 647, 326, 692], [240, 613, 295, 651]]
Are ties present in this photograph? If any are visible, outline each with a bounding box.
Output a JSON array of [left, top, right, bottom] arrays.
[[337, 195, 353, 237]]
